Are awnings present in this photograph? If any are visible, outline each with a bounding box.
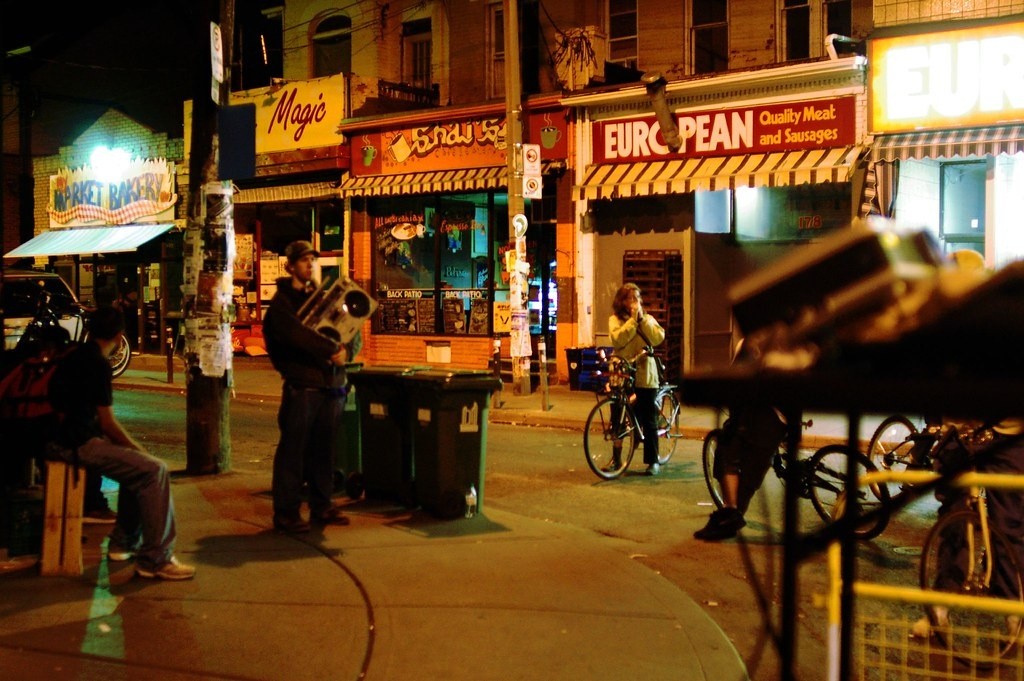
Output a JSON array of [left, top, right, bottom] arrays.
[[3, 223, 175, 258], [575, 148, 863, 201], [871, 123, 1024, 161], [340, 163, 550, 198]]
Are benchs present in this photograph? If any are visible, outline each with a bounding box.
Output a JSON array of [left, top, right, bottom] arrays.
[[40, 457, 86, 572]]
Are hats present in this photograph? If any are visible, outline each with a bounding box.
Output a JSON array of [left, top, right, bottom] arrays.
[[285, 240, 320, 275]]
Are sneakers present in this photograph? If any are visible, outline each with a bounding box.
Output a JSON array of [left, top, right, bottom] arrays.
[[108, 539, 140, 562], [134, 556, 196, 581]]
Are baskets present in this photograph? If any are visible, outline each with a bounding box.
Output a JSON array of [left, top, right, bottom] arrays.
[[588, 372, 629, 396]]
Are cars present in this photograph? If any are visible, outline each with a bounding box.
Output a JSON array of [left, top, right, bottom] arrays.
[[0, 272, 89, 352]]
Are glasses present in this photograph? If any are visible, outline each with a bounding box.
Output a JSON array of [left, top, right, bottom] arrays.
[[626, 296, 643, 301]]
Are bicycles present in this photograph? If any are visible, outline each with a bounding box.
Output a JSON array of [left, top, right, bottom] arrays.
[[701, 402, 891, 541], [866, 414, 1024, 670], [569, 344, 682, 480]]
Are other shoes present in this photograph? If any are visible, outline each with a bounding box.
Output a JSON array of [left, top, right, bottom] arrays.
[[309, 508, 350, 526], [693, 509, 746, 541], [273, 517, 312, 535]]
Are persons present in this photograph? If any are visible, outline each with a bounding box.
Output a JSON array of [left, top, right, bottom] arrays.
[[694, 338, 787, 541], [903, 501, 1024, 638], [601, 283, 664, 477], [0, 327, 118, 524], [48, 306, 197, 583], [262, 241, 361, 541]]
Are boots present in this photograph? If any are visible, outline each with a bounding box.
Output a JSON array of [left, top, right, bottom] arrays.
[[646, 463, 661, 475], [600, 446, 623, 472]]
